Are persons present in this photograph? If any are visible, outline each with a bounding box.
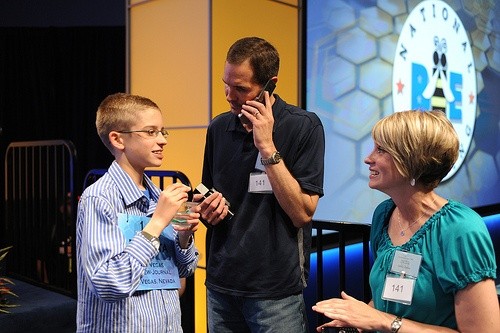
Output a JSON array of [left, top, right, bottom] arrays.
[[192, 36, 325, 333], [76, 93, 201, 333], [312, 110, 500, 333]]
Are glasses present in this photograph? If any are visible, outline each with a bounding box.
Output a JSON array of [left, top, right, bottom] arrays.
[[110, 129, 169, 139]]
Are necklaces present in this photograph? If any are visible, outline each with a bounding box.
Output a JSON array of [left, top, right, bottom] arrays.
[[398, 197, 437, 236]]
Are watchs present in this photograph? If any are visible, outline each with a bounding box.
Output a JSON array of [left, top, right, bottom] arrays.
[[391, 316, 403, 333], [260, 151, 281, 166], [141, 231, 160, 251]]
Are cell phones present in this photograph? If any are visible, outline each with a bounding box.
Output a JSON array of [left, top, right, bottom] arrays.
[[238, 79, 277, 124]]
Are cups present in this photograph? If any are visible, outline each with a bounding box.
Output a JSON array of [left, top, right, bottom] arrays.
[[170, 202, 199, 230]]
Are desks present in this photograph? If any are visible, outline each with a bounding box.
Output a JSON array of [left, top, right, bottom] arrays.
[[0, 276, 77, 333]]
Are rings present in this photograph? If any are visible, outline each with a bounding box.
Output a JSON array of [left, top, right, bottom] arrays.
[[253, 111, 259, 116]]
